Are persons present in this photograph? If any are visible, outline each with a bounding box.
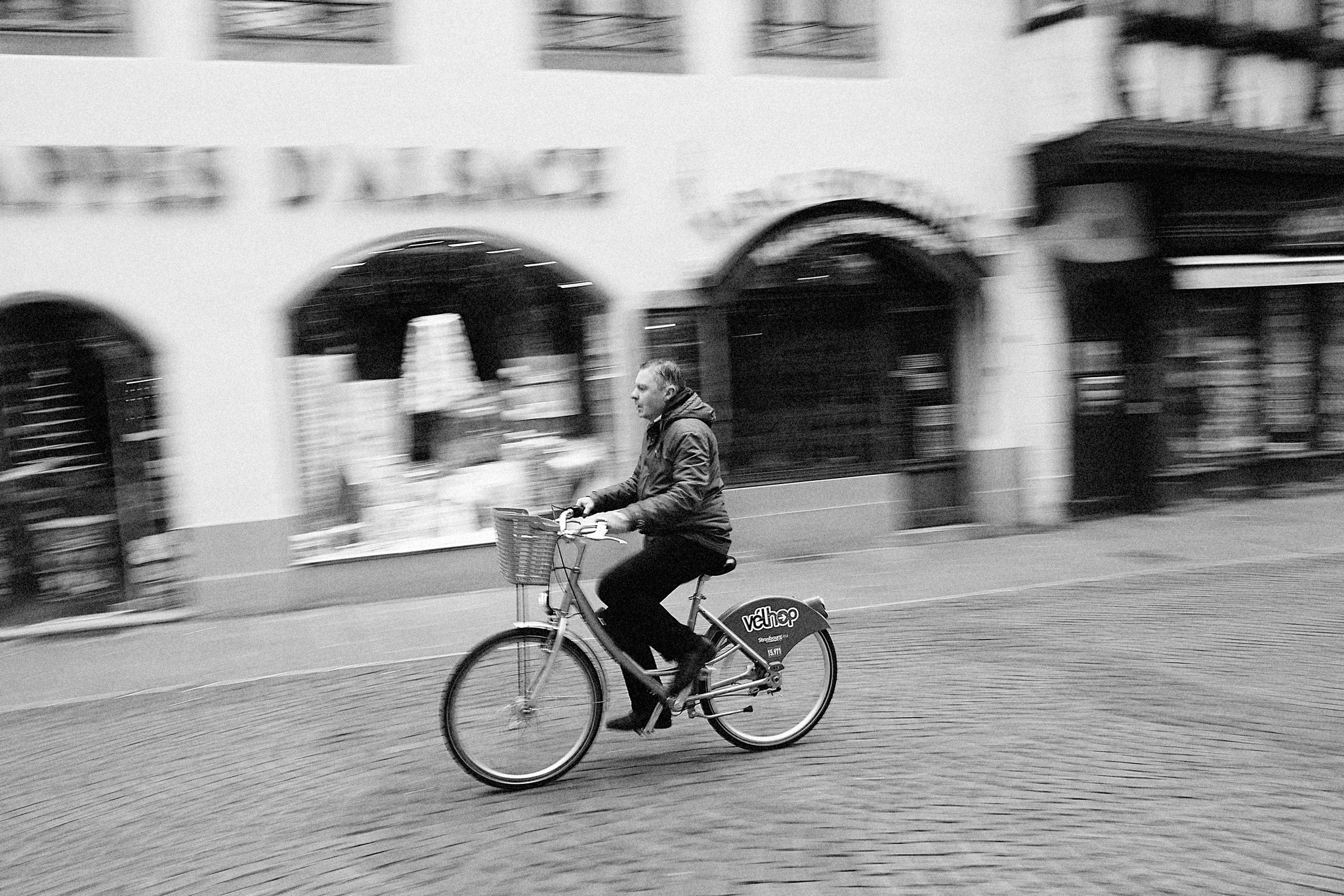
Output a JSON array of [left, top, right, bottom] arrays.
[[575, 356, 734, 731]]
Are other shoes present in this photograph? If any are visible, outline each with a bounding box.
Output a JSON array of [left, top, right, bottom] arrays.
[[603, 711, 671, 731], [664, 637, 718, 698]]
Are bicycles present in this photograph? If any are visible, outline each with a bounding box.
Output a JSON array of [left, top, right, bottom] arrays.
[[436, 506, 845, 792]]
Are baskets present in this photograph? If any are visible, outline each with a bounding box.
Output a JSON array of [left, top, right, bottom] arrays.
[[491, 507, 560, 585]]
